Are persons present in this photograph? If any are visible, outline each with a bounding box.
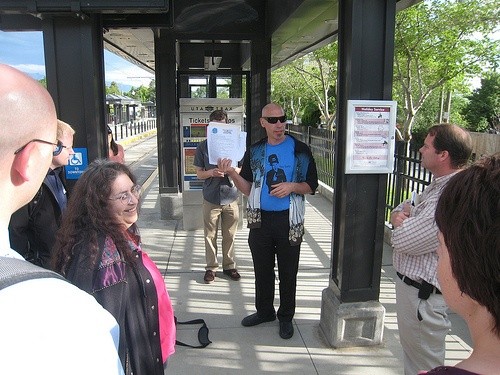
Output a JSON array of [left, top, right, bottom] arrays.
[[193, 110, 244, 283], [390, 123, 473, 375], [53, 160, 176, 375], [217, 103, 319, 339], [417, 152, 500, 375], [107, 125, 124, 166], [0, 64, 125, 375], [8, 120, 75, 279]]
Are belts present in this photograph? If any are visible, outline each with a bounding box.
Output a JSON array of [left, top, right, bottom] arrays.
[[396, 271, 441, 294]]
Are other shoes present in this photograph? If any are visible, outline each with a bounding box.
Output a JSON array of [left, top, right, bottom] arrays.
[[223, 269, 241, 282], [279, 317, 295, 340], [204, 269, 216, 284], [240, 307, 277, 328]]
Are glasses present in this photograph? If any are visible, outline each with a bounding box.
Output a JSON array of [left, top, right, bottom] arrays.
[[12, 139, 67, 156], [260, 116, 288, 124], [106, 185, 143, 205]]
